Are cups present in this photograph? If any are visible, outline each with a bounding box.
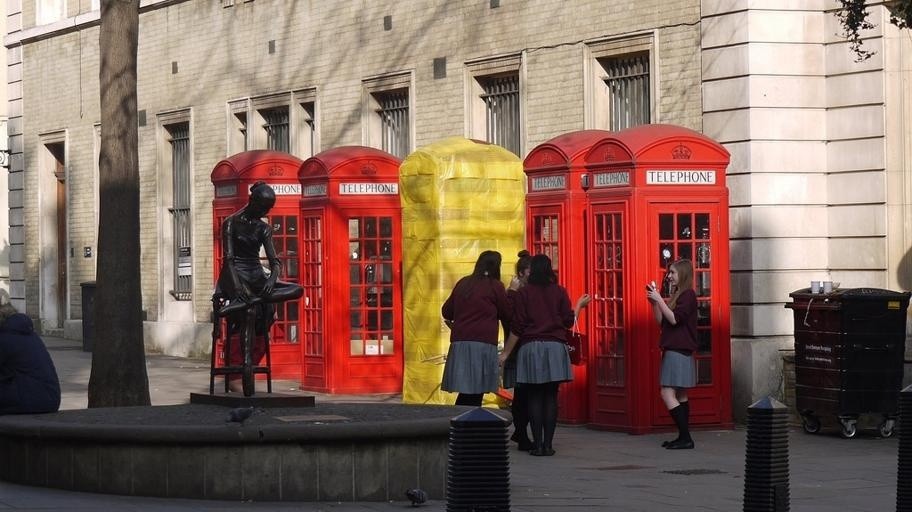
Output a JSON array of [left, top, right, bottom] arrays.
[[824, 281, 832, 293], [812, 280, 820, 293]]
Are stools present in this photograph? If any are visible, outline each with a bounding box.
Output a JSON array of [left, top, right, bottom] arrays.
[[206, 293, 276, 397]]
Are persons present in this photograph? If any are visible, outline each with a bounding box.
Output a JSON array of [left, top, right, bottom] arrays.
[[441, 251, 520, 406], [647, 259, 698, 449], [501, 250, 535, 450], [499, 254, 591, 456], [0, 288, 61, 415], [218, 181, 305, 396]]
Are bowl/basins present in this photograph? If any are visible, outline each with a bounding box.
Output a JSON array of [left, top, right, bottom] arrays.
[[831, 280, 839, 290]]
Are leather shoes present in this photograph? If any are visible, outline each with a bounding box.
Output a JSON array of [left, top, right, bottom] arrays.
[[660, 439, 694, 449], [511, 428, 555, 456]]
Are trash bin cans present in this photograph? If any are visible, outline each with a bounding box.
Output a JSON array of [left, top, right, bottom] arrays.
[[80, 281, 98, 350], [783, 356, 803, 426]]
